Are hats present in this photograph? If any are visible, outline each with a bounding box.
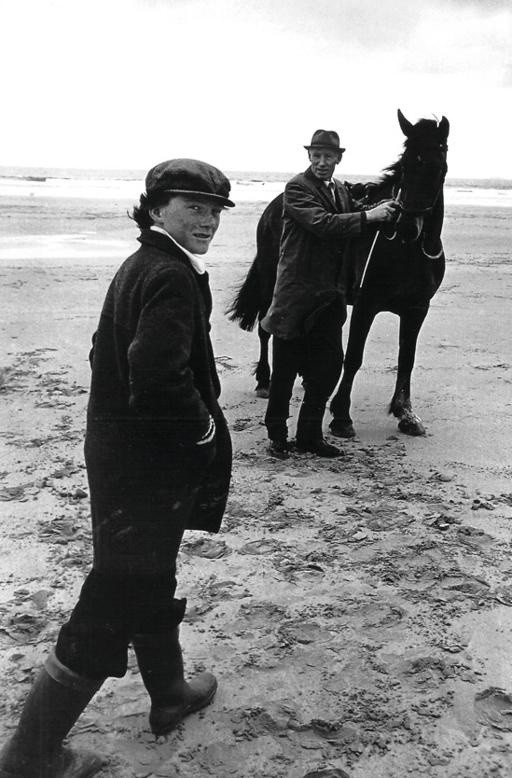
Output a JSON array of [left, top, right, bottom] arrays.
[[144, 158, 235, 207], [304, 130, 346, 153]]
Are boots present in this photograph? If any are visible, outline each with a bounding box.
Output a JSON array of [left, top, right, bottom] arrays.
[[130, 623, 217, 735], [1, 648, 105, 778]]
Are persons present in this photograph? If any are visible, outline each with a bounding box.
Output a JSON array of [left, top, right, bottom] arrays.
[[257, 130, 408, 457], [0, 159, 239, 776]]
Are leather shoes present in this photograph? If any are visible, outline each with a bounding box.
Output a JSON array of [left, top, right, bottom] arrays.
[[269, 436, 289, 458], [296, 435, 340, 457]]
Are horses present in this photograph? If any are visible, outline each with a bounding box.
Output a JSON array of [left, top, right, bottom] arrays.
[[221, 105, 451, 441]]
